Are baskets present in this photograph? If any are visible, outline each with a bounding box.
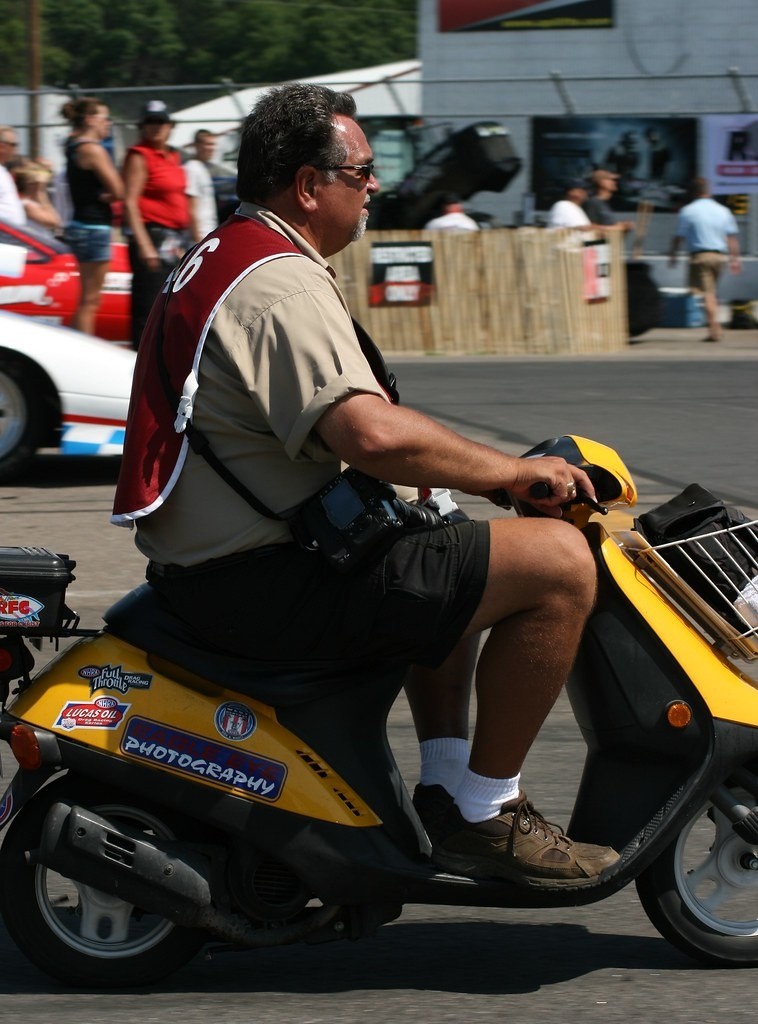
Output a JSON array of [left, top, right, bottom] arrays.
[[621, 519, 758, 664]]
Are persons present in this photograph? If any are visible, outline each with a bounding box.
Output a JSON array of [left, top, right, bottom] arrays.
[[549, 168, 635, 234], [0, 98, 218, 350], [425, 192, 477, 234], [111, 82, 622, 886], [665, 177, 743, 342]]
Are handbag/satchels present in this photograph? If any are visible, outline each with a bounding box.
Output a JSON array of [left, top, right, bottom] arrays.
[[283, 462, 409, 576], [633, 483, 758, 637]]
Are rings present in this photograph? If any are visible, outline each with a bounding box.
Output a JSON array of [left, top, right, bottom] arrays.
[[566, 481, 576, 490]]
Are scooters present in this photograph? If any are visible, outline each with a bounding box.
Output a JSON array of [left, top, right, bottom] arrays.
[[1, 435, 758, 993]]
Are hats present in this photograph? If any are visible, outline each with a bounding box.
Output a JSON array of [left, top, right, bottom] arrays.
[[134, 100, 178, 128], [565, 177, 591, 190], [588, 169, 620, 182]]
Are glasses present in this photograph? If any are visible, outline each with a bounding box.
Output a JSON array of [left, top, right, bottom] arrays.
[[290, 162, 374, 181]]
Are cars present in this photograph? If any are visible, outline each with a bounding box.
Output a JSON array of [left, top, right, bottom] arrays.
[[0, 302, 143, 488], [1, 218, 135, 352]]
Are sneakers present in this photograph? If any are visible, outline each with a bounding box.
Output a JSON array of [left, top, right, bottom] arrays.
[[412, 781, 620, 887]]
